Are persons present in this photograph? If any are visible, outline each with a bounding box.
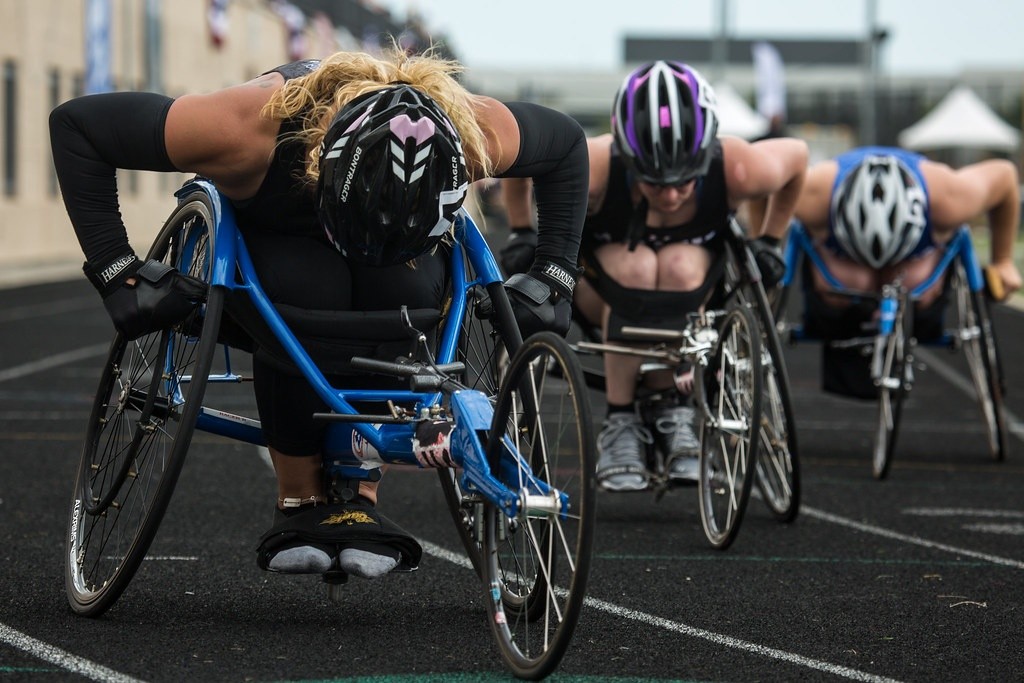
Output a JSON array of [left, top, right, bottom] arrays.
[[735, 145, 1024, 402], [500, 58, 809, 491], [48, 29, 589, 580]]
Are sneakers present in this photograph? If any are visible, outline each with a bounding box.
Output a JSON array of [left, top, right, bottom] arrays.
[[595, 413, 650, 490], [654, 407, 714, 481]]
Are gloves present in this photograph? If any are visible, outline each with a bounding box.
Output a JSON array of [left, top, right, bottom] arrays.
[[474, 257, 583, 361], [85, 249, 208, 342], [501, 226, 540, 277], [753, 232, 785, 286]]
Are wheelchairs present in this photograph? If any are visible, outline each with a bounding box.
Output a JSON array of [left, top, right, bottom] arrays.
[[494, 249, 804, 555], [64, 178, 601, 681], [766, 211, 1010, 484]]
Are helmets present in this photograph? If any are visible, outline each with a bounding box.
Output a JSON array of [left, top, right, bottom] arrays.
[[317, 86, 468, 268], [610, 60, 718, 184], [831, 154, 928, 271]]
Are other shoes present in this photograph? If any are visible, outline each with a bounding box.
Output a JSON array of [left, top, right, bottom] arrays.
[[258, 505, 401, 579]]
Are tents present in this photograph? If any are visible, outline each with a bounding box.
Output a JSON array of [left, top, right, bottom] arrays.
[[899, 83, 1022, 161], [709, 81, 771, 143]]
